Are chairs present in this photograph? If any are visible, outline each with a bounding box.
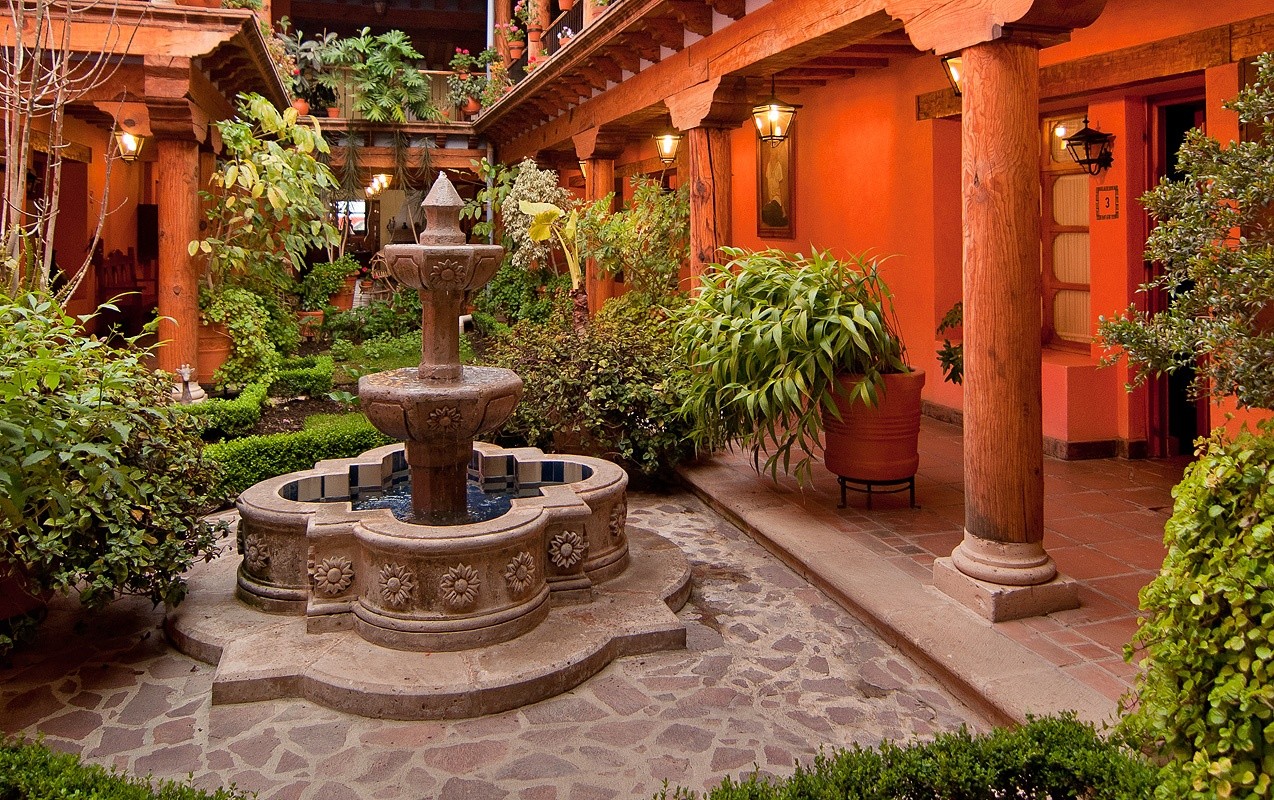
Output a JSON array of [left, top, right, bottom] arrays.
[[91, 239, 145, 340]]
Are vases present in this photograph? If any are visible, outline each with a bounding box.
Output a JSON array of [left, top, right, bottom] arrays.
[[591, 6, 609, 18], [536, 55, 549, 68], [490, 65, 503, 79], [558, 0, 574, 11], [459, 66, 469, 79], [559, 38, 571, 48], [461, 94, 481, 115], [439, 110, 450, 119], [361, 278, 372, 289], [508, 41, 525, 59], [527, 25, 543, 42]]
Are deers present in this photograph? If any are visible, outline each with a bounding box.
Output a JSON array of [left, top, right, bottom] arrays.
[[175, 364, 195, 406]]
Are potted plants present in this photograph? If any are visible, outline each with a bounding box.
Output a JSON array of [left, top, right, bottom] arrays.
[[308, 28, 346, 117], [293, 270, 329, 336], [277, 25, 315, 115], [196, 91, 342, 385], [323, 256, 361, 312], [665, 246, 929, 514]]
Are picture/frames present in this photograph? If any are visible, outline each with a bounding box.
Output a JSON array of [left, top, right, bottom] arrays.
[[757, 119, 798, 240]]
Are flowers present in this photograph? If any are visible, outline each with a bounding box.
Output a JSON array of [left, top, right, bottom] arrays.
[[437, 0, 612, 120], [363, 266, 372, 281]]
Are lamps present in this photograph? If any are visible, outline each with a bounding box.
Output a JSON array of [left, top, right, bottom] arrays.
[[578, 160, 587, 179], [108, 130, 146, 163], [751, 73, 804, 148], [650, 112, 685, 168], [364, 175, 391, 200], [940, 53, 965, 93], [1063, 113, 1115, 175], [387, 215, 396, 237]]
